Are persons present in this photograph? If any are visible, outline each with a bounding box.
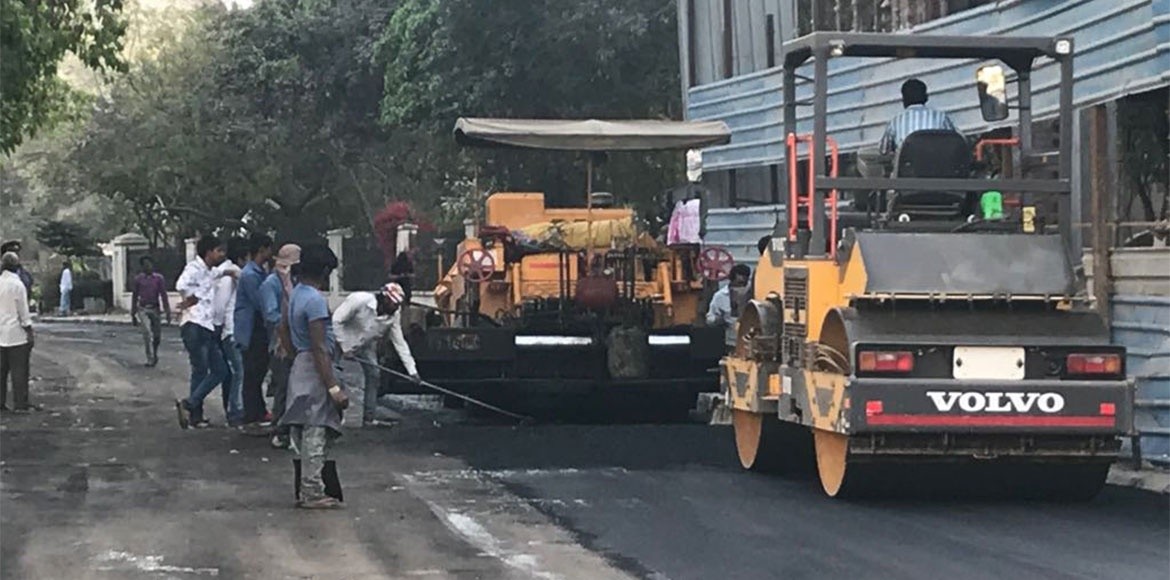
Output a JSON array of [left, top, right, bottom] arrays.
[[175, 232, 419, 447], [879, 79, 956, 178], [705, 264, 751, 351], [279, 242, 347, 508], [0, 240, 40, 414], [132, 257, 171, 367], [752, 235, 773, 293], [56, 262, 73, 316]]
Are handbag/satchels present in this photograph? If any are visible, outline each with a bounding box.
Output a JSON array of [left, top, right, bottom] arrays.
[[293, 460, 344, 502]]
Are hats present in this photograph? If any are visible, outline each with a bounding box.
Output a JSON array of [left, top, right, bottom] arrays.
[[1, 241, 21, 251], [381, 282, 405, 304], [275, 243, 302, 265], [300, 244, 339, 267]]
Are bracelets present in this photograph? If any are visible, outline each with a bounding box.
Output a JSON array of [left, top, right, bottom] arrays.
[[327, 385, 340, 395]]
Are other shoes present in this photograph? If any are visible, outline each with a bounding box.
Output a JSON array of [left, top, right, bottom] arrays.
[[0, 406, 10, 412], [363, 420, 393, 428], [16, 405, 43, 413], [177, 399, 191, 429], [196, 420, 211, 427], [257, 412, 275, 426], [144, 359, 159, 366], [295, 497, 348, 510], [225, 423, 246, 431], [271, 436, 288, 446], [239, 424, 273, 437]]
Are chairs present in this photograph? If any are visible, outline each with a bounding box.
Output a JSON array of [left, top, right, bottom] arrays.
[[898, 128, 971, 219]]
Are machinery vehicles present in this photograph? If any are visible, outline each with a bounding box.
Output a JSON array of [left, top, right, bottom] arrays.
[[372, 116, 730, 430], [720, 30, 1141, 506]]
